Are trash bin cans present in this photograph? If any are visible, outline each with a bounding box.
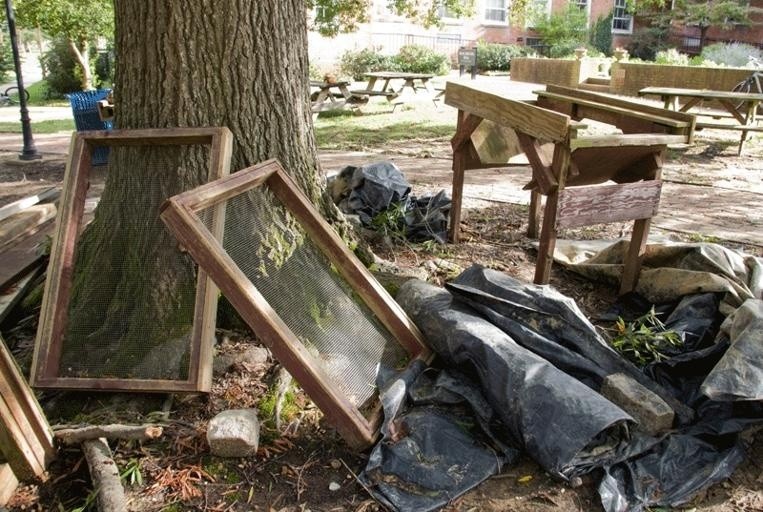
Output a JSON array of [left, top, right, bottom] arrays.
[[64, 89, 114, 165]]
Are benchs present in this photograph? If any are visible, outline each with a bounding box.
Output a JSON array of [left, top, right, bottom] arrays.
[[697, 121, 763, 156], [331, 92, 370, 111]]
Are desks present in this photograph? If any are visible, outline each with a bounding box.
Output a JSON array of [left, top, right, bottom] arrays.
[[363, 70, 433, 95], [417, 85, 446, 105], [641, 82, 763, 154], [309, 77, 351, 102], [348, 91, 398, 107], [690, 110, 763, 122]]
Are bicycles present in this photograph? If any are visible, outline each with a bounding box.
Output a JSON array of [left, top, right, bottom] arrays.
[[0, 86, 30, 104], [729, 55, 763, 110]]
